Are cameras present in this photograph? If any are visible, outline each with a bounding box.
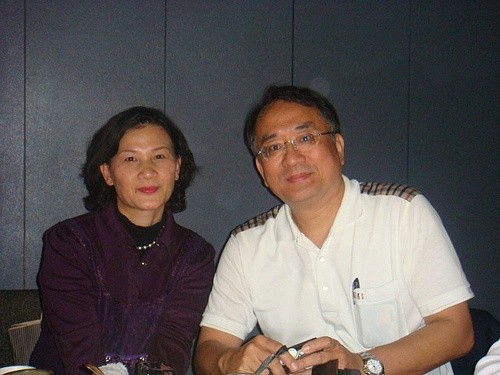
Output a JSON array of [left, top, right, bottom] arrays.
[[279, 337, 322, 375]]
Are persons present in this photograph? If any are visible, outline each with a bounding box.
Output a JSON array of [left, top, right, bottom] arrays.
[[192, 84, 475, 375], [29, 105, 217, 375]]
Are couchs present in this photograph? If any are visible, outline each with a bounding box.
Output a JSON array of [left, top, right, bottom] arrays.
[[0, 288, 500, 375]]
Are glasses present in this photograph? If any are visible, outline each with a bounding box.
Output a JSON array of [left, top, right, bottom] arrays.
[[256, 131, 336, 158]]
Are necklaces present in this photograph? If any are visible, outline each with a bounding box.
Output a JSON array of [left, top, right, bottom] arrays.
[[135, 225, 164, 250]]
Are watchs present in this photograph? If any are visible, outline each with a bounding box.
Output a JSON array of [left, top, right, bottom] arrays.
[[359, 351, 385, 375]]
